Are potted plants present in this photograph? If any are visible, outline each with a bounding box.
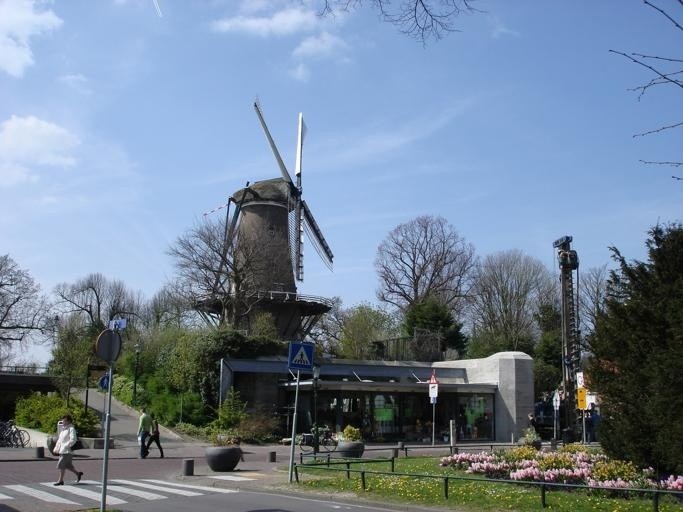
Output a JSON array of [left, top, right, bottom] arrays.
[[338, 425, 364, 457], [205, 434, 242, 471], [216, 426, 241, 444], [46, 428, 60, 456], [517, 425, 541, 451]]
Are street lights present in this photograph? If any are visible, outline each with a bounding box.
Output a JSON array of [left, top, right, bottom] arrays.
[[310, 360, 319, 454], [131, 341, 140, 405]]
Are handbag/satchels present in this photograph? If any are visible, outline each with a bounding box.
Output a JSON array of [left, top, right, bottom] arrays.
[[70, 439, 83, 450]]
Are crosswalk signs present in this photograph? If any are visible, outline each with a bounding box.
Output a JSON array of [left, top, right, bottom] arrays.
[[287, 340, 314, 370]]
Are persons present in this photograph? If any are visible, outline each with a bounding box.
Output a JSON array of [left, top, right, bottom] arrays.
[[135, 407, 153, 459], [415, 418, 433, 444], [144, 410, 164, 458], [51, 414, 83, 486], [526, 412, 536, 427]]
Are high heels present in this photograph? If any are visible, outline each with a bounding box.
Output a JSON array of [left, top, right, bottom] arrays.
[[77, 472, 83, 481], [54, 481, 63, 485]]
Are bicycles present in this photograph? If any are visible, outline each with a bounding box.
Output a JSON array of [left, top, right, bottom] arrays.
[[298, 424, 336, 452], [0, 419, 29, 447]]
